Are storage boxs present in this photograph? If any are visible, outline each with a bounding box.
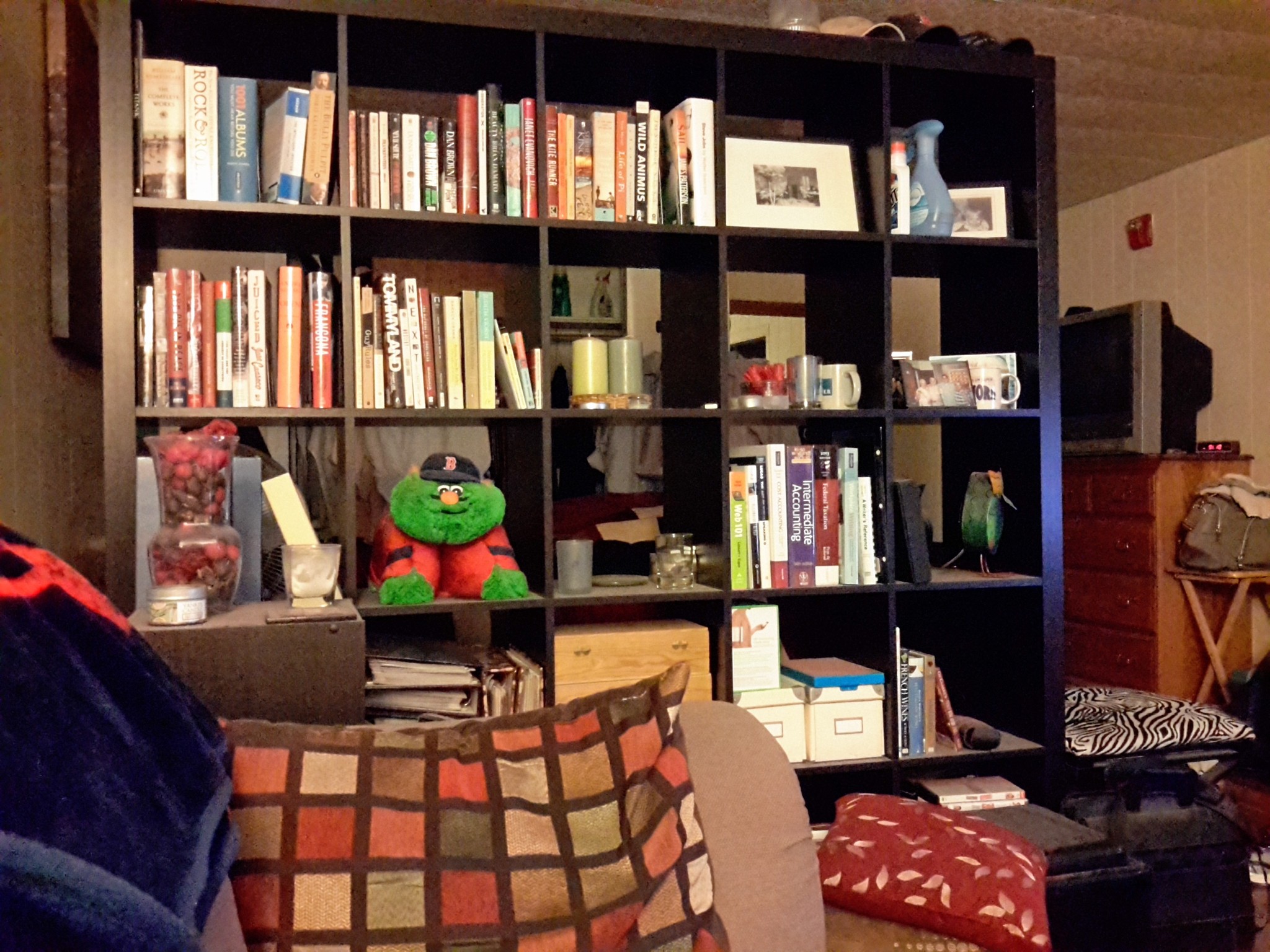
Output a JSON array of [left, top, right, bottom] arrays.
[[785, 652, 885, 759], [555, 622, 715, 711], [731, 672, 808, 765]]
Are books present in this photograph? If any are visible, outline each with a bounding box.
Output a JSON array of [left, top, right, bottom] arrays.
[[132, 53, 719, 409], [895, 623, 1030, 813], [727, 441, 933, 591], [365, 637, 546, 727]]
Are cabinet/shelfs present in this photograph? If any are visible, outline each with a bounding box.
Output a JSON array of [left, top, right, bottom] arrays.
[[100, 0, 1073, 814], [1060, 449, 1253, 701]]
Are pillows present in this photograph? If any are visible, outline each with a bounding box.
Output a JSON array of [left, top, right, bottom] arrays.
[[814, 793, 1053, 952], [1067, 688, 1256, 760], [215, 655, 732, 952]]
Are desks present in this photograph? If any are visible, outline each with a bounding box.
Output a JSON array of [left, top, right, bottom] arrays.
[[1165, 566, 1269, 705]]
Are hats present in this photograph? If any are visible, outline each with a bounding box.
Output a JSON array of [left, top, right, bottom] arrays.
[[419, 454, 483, 484]]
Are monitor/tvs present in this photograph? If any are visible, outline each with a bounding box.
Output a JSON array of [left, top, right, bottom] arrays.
[[1060, 301, 1215, 452]]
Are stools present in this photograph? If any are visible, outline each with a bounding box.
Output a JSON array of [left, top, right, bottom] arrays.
[[1077, 806, 1256, 951], [953, 802, 1156, 952]]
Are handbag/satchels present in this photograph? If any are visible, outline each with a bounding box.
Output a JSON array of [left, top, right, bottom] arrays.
[[1176, 473, 1270, 572]]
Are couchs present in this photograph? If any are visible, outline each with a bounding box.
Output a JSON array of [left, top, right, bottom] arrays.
[[0, 525, 826, 952]]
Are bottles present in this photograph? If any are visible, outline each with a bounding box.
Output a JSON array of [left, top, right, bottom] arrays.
[[142, 433, 242, 614]]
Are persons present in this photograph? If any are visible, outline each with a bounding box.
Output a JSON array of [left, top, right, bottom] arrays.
[[954, 380, 971, 408], [953, 207, 989, 234], [926, 377, 943, 407], [915, 378, 932, 407], [951, 197, 989, 232], [937, 374, 964, 407]]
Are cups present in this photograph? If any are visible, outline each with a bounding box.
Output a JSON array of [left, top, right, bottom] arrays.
[[787, 356, 822, 410], [282, 543, 344, 609], [970, 368, 1022, 409], [655, 533, 696, 592], [556, 539, 594, 596], [819, 364, 860, 410]]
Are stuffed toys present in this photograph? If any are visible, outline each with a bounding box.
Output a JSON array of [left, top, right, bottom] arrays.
[[366, 453, 530, 607]]
